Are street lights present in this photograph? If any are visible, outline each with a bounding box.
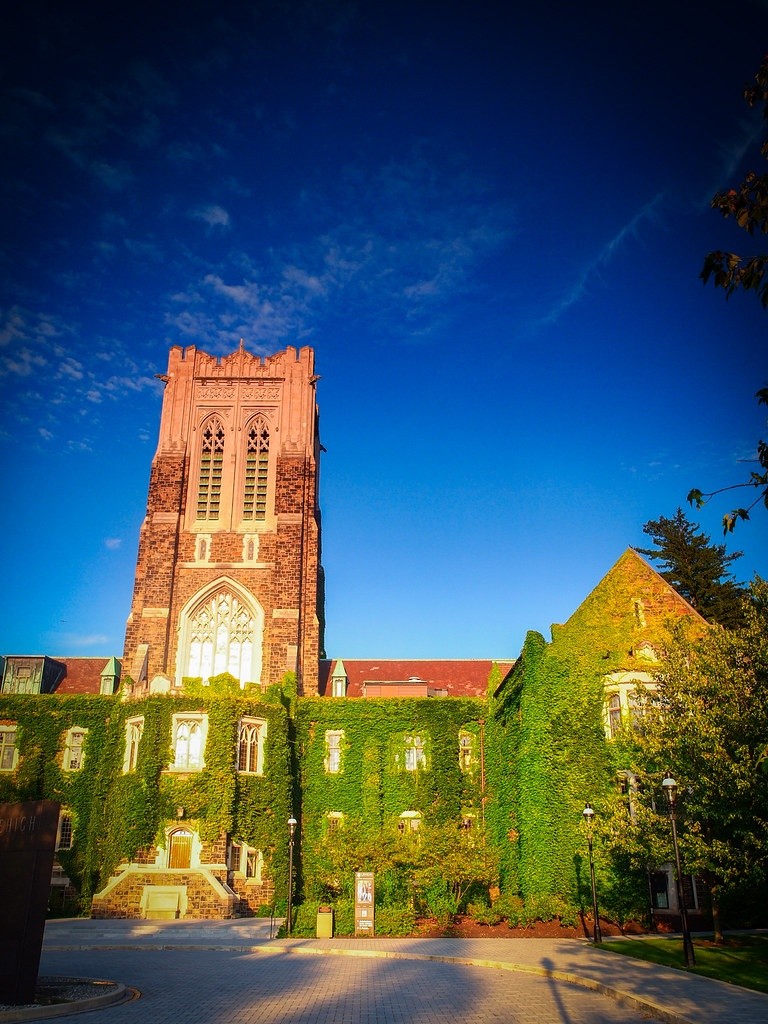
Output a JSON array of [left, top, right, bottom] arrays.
[[287, 814, 297, 933], [583, 803, 602, 944], [662, 772, 695, 968]]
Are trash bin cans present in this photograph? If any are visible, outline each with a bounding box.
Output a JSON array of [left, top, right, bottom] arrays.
[[316, 906, 333, 938]]
